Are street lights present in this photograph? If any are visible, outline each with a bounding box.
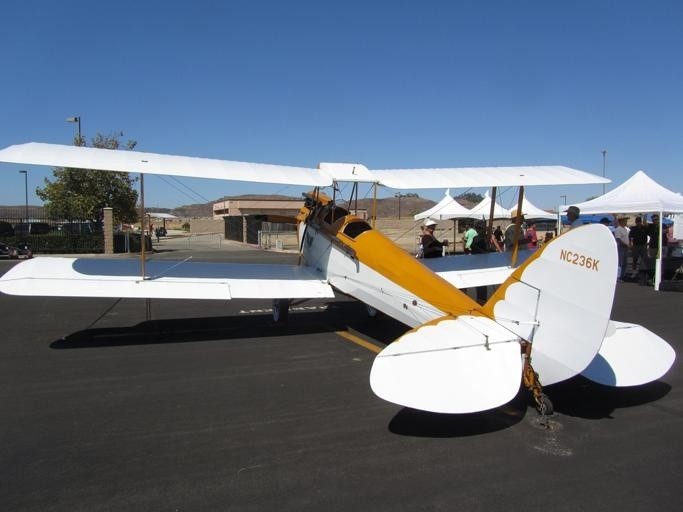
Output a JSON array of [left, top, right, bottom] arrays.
[[20, 171, 29, 231], [66, 117, 80, 146]]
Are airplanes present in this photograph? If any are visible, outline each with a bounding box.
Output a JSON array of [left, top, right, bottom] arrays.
[[0, 143, 677, 415]]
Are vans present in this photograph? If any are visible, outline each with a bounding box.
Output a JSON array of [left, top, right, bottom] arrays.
[[1, 219, 96, 237]]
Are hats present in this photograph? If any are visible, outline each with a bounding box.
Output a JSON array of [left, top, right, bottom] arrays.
[[425, 221, 438, 227], [564, 206, 579, 214], [511, 210, 526, 218]]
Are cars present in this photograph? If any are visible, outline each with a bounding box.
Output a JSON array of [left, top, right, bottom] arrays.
[[0, 243, 18, 260]]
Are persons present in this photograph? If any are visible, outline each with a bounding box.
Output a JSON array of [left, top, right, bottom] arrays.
[[470, 220, 492, 304], [423, 220, 448, 258], [629, 216, 649, 279], [600, 217, 615, 237], [503, 209, 532, 250], [615, 217, 630, 283], [563, 205, 583, 228], [494, 226, 504, 242], [645, 215, 669, 257], [524, 221, 538, 249], [465, 221, 478, 255]]
